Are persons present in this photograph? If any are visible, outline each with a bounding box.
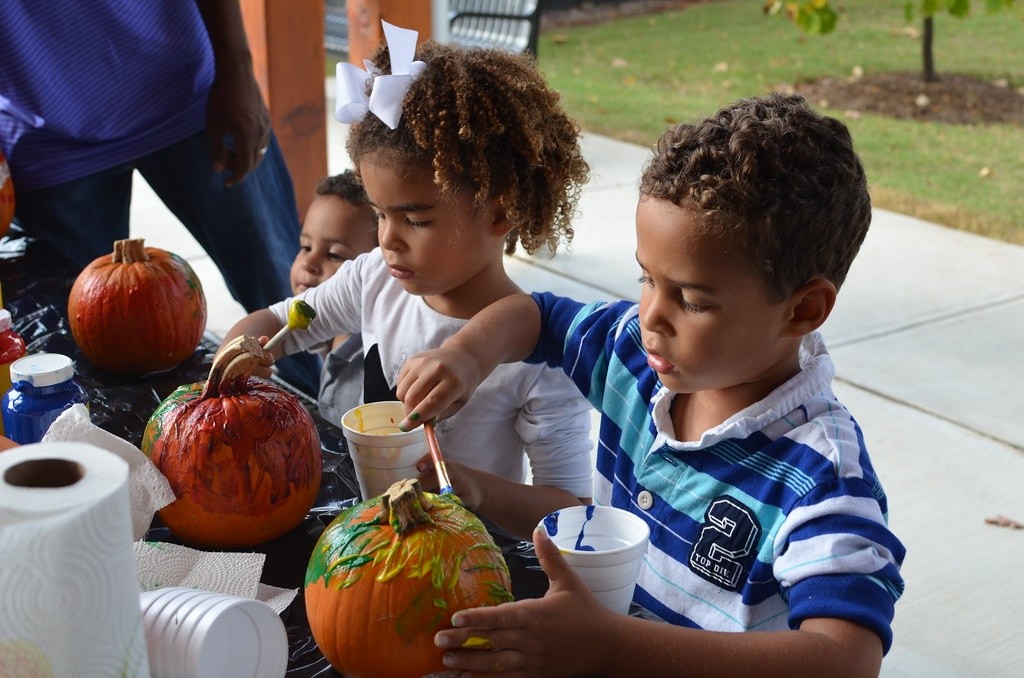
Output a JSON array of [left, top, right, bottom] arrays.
[[214, 19, 595, 543], [396, 91, 907, 678], [0, 0, 324, 401]]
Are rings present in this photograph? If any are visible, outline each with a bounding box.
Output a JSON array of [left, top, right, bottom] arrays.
[[260, 146, 268, 155]]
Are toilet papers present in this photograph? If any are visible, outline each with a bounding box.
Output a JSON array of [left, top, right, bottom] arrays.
[[0, 441, 152, 676]]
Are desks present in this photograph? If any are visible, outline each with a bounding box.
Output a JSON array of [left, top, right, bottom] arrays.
[[0, 224, 708, 676]]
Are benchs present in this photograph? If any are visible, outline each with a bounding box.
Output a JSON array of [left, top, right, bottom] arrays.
[[323, 0, 540, 60]]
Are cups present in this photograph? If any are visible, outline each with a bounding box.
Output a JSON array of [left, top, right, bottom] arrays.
[[538, 505, 650, 616], [139, 583, 289, 678], [340, 401, 435, 501]]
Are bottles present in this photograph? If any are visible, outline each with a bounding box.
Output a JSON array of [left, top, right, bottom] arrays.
[[0, 309, 26, 436], [2, 353, 90, 445]]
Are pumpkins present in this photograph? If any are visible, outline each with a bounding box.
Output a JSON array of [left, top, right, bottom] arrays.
[[67, 236, 207, 372], [139, 334, 323, 551], [305, 478, 513, 678]]
